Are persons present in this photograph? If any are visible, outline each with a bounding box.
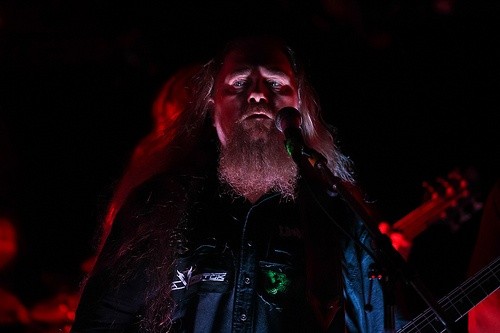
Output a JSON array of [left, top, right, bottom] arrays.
[[0, 219, 46, 332], [69, 38, 388, 333]]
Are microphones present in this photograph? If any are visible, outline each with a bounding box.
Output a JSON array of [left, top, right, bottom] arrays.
[[274, 105, 309, 163]]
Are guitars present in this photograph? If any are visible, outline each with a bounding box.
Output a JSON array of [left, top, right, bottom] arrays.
[[394, 171, 482, 240], [392, 256, 500, 333]]
